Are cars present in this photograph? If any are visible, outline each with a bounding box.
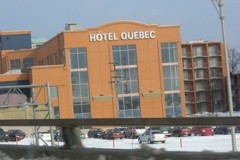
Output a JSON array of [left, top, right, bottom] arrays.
[[0, 124, 240, 145]]
